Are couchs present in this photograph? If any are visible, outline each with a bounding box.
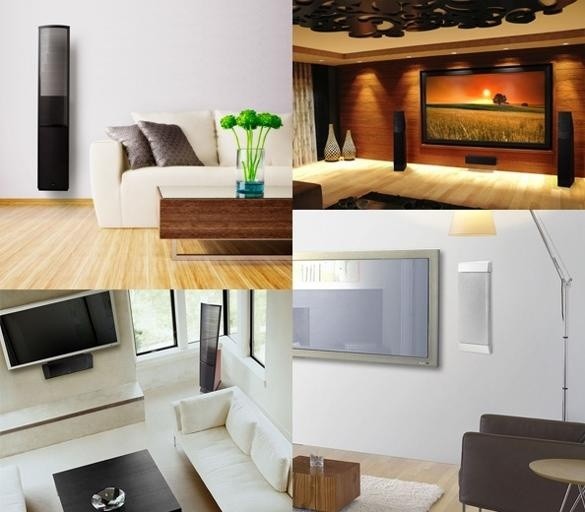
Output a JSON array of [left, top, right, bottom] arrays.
[[172, 385, 292, 512], [0, 465, 28, 512], [89, 110, 293, 231]]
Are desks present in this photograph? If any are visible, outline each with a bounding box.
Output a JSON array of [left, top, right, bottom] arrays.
[[160, 198, 292, 263]]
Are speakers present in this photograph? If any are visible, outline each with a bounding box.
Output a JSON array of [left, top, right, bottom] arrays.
[[557, 111, 575, 188], [37, 24, 70, 191], [392, 110, 407, 172]]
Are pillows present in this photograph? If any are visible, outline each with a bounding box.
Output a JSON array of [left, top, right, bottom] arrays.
[[140, 121, 203, 165], [105, 125, 152, 169]]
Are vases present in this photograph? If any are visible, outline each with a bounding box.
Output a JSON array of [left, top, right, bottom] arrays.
[[343, 130, 356, 161], [324, 123, 339, 161]]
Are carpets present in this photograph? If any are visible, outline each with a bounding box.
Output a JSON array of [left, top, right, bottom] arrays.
[[292, 476, 446, 512]]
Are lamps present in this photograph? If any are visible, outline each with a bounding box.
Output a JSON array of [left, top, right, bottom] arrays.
[[452, 210, 572, 424]]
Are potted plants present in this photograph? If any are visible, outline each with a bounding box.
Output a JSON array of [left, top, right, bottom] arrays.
[[220, 110, 285, 197]]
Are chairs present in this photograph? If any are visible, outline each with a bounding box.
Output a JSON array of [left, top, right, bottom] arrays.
[[456, 412, 585, 512]]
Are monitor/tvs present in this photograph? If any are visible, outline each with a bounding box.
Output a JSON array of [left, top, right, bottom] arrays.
[[291, 248, 440, 370], [0, 288, 121, 379], [419, 62, 554, 153]]
